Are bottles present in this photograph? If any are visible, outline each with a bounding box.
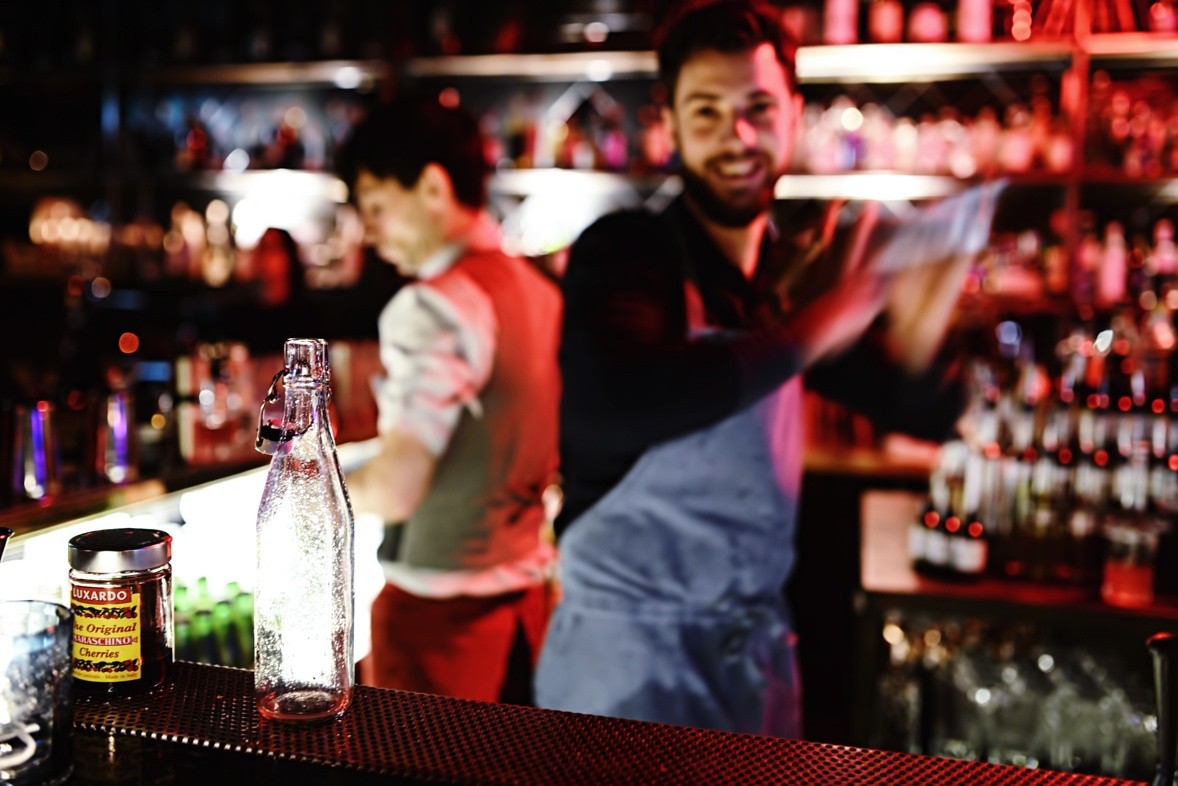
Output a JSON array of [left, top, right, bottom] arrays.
[[907, 392, 1178, 601], [178, 577, 255, 668], [254, 338, 354, 725], [66, 529, 176, 709]]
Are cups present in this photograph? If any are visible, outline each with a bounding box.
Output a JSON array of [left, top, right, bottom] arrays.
[[1, 601, 73, 786]]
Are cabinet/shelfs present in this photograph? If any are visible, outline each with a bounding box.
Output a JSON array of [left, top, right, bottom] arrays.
[[0, 0, 1178, 476]]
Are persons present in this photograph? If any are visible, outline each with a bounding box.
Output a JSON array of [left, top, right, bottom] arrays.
[[225, 224, 322, 353], [328, 104, 563, 708], [533, 5, 986, 732]]
